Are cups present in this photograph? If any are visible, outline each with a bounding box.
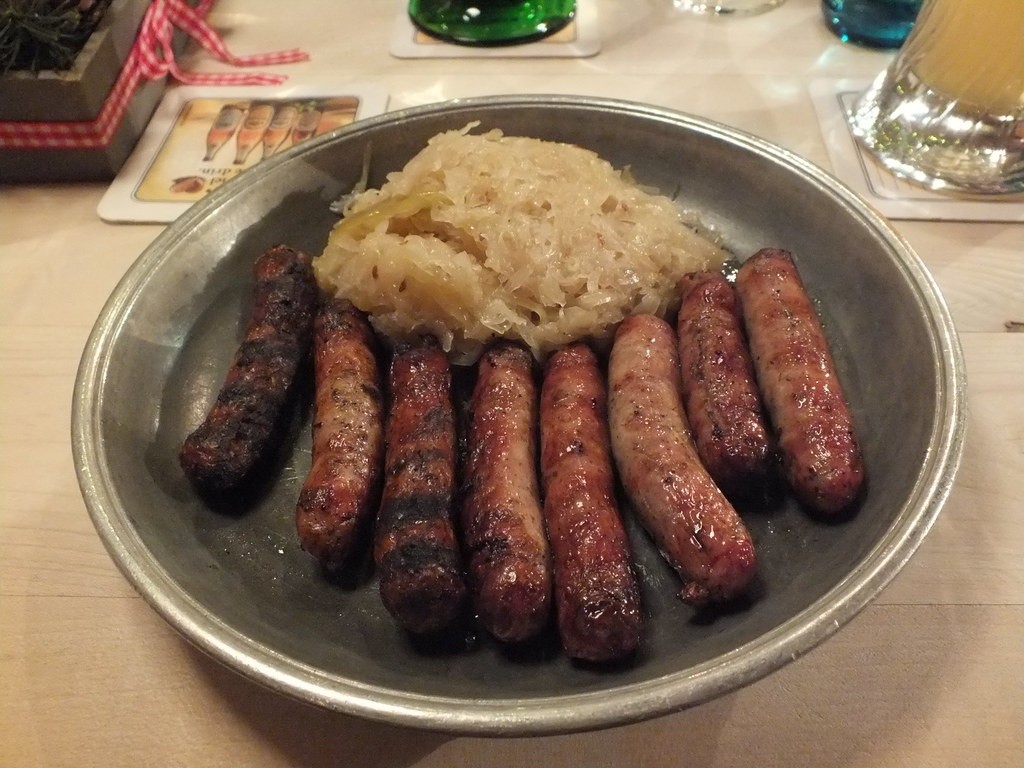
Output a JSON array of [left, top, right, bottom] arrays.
[[672, 0, 789, 20], [851, 0, 1024, 201], [408, 0, 578, 48], [820, 0, 923, 50]]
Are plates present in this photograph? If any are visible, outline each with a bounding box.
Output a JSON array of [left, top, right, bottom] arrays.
[[67, 91, 969, 735]]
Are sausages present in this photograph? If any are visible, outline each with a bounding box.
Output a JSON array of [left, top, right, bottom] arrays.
[[174, 246, 865, 665]]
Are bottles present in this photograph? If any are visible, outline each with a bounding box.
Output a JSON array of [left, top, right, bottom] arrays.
[[203, 101, 324, 165]]
